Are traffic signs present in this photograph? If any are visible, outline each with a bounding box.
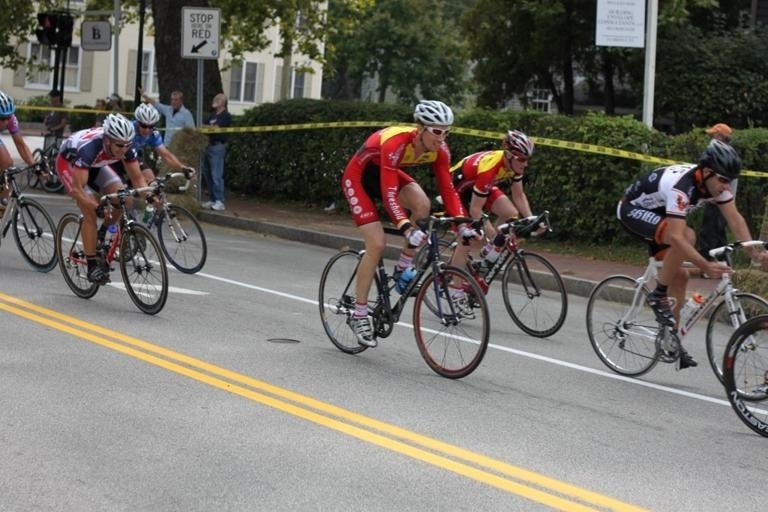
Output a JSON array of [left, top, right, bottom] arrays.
[[179, 5, 222, 62]]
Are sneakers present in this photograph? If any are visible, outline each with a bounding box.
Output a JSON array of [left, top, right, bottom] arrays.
[[646, 293, 677, 326], [212, 200, 226, 211], [202, 201, 213, 209], [346, 315, 378, 349], [450, 295, 477, 320], [394, 265, 422, 297], [86, 267, 109, 284]]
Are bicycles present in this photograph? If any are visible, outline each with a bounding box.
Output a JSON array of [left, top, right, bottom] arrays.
[[0, 128, 207, 316]]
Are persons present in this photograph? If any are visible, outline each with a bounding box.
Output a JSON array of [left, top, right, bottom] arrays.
[[38, 84, 197, 230], [699, 122, 739, 280], [56, 112, 164, 284], [0, 91, 50, 220], [339, 98, 483, 350], [198, 92, 230, 211], [439, 125, 549, 320], [615, 137, 766, 372]]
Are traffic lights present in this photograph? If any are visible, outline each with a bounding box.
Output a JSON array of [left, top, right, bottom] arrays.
[[32, 9, 58, 44]]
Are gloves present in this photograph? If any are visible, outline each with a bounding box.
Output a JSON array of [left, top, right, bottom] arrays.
[[406, 228, 427, 247]]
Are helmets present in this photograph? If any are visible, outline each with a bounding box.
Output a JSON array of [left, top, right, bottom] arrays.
[[699, 138, 741, 179], [132, 103, 161, 127], [412, 99, 455, 127], [101, 113, 137, 143], [0, 92, 15, 117], [503, 130, 535, 156]]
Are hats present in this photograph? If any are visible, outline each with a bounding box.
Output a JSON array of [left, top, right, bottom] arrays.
[[49, 90, 59, 97], [705, 123, 732, 137], [106, 95, 119, 101]]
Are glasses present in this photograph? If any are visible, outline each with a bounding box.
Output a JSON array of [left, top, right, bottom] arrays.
[[424, 125, 449, 137], [504, 149, 531, 162], [114, 142, 132, 148], [710, 170, 732, 184], [139, 122, 154, 129]]
